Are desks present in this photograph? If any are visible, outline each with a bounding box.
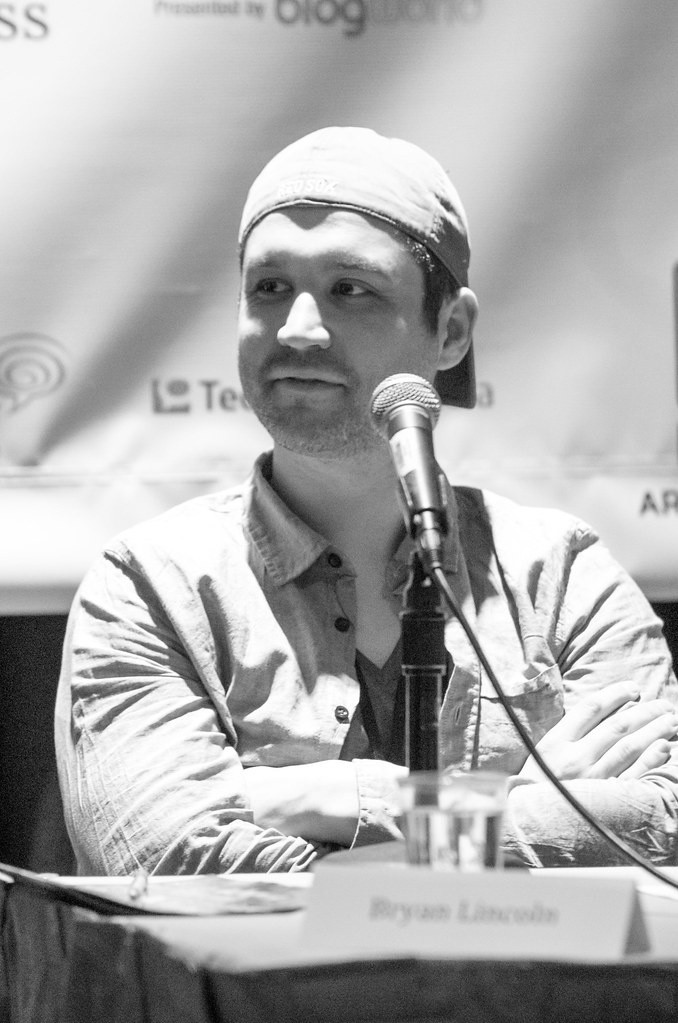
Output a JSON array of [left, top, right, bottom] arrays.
[[0, 862, 678, 1023]]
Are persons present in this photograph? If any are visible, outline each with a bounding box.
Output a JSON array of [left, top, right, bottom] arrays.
[[53, 126, 678, 876]]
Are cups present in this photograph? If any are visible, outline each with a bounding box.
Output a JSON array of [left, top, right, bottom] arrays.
[[397, 768, 511, 873]]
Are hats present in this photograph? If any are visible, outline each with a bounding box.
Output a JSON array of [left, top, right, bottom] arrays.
[[239, 126, 478, 409]]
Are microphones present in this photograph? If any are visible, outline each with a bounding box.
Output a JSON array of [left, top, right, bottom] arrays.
[[368, 373, 446, 630]]
